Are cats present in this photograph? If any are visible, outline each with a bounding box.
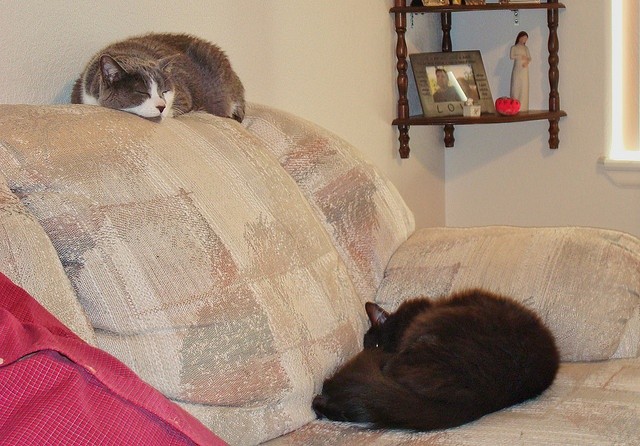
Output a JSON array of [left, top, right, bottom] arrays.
[[310, 288, 563, 436], [69, 31, 247, 124]]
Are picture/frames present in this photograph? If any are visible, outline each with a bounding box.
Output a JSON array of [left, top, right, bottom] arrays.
[[408, 49, 497, 117]]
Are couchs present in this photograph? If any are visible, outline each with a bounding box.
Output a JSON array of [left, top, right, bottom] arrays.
[[2, 101, 640, 446]]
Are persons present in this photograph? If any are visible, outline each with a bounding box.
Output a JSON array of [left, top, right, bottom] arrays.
[[433, 69, 461, 102], [511, 30, 532, 110]]
